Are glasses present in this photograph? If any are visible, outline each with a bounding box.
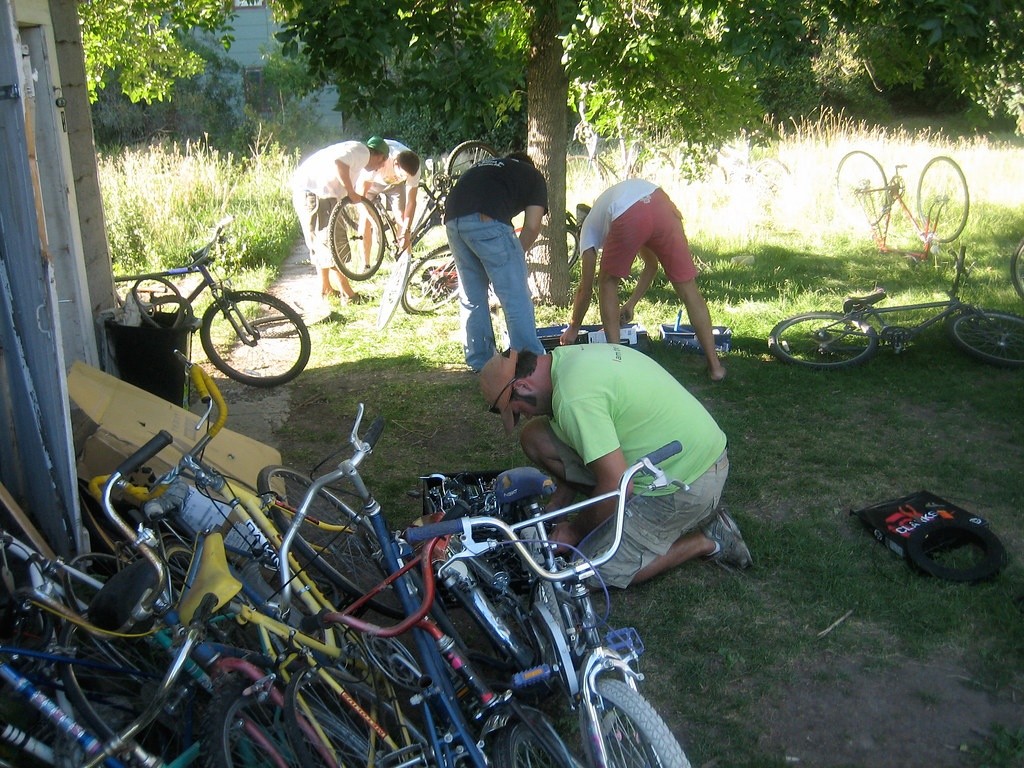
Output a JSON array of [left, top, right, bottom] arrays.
[[489, 377, 518, 413]]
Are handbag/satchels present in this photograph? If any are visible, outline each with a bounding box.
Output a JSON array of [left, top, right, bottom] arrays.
[[849, 490, 1007, 582]]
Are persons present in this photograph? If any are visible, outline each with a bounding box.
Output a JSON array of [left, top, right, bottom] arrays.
[[444, 153, 548, 372], [559, 177, 726, 381], [291, 136, 391, 306], [481, 343, 753, 592], [355, 139, 422, 273]]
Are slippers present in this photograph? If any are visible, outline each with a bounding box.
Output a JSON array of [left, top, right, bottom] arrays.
[[707, 366, 726, 386]]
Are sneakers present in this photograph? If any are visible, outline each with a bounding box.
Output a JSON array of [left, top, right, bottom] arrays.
[[700, 510, 752, 567]]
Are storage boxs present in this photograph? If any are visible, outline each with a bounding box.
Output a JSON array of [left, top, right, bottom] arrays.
[[660, 324, 731, 355], [536, 322, 637, 349], [63, 359, 294, 532]]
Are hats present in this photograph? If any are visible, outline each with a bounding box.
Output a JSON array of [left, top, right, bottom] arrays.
[[478, 349, 520, 436], [367, 135, 390, 158]]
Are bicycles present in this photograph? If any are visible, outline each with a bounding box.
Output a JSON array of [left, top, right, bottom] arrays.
[[401, 204, 593, 316], [1, 364, 687, 767], [113, 215, 312, 387], [767, 245, 1023, 371], [328, 139, 499, 282], [836, 149, 972, 271]]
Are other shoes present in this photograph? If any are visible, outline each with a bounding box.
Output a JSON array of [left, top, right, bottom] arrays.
[[346, 292, 374, 307], [322, 290, 344, 301]]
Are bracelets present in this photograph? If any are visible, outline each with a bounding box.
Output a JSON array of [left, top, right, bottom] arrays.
[[569, 318, 581, 328], [406, 226, 411, 233]]
